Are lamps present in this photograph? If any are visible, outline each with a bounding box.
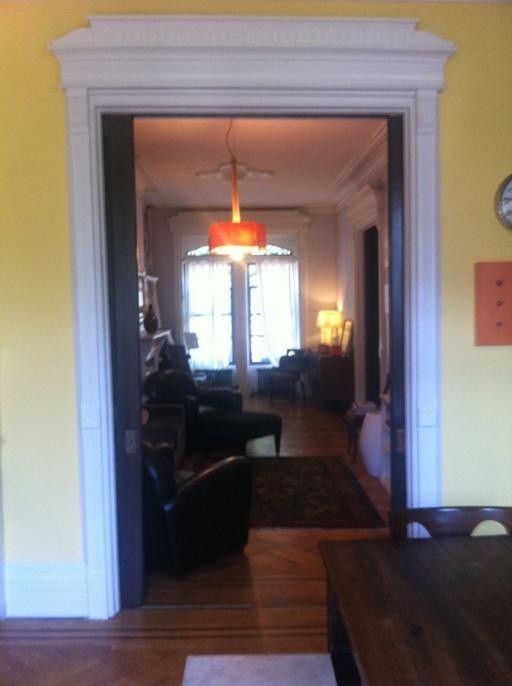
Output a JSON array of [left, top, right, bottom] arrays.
[[317, 310, 343, 346], [206, 160, 268, 257]]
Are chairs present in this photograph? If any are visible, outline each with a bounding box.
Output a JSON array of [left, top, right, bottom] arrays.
[[142, 369, 242, 467], [269, 349, 309, 406], [142, 446, 253, 577], [389, 505, 511, 537]]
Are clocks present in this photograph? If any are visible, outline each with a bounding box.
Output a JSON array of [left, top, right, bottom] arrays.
[[494, 174, 512, 233]]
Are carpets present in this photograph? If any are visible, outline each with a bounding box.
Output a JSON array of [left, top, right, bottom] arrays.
[[249, 454, 386, 529], [181, 652, 339, 685]]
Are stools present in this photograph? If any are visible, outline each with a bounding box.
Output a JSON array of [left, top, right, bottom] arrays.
[[200, 412, 282, 454]]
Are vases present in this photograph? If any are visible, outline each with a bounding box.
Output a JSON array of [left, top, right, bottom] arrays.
[[144, 303, 158, 334]]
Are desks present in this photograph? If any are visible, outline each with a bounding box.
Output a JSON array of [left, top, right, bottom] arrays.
[[318, 535, 512, 685], [306, 353, 354, 418]]
